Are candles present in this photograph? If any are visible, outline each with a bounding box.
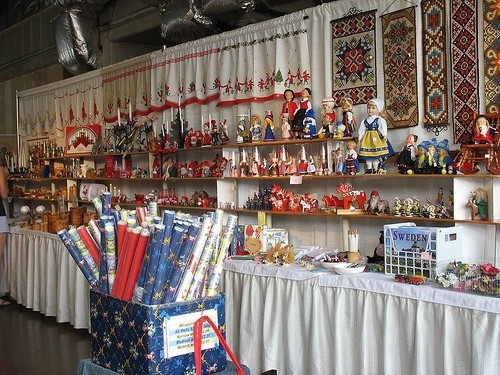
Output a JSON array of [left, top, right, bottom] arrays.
[[117, 101, 211, 136]]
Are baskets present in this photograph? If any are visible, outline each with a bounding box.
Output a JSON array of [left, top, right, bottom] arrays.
[[70, 206, 87, 229], [48, 211, 69, 233]]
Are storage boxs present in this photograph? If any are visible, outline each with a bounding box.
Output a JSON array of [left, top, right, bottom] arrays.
[[91, 288, 227, 375], [383, 223, 465, 281]]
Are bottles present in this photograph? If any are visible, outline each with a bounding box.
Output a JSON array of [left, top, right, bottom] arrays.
[[33, 140, 64, 157]]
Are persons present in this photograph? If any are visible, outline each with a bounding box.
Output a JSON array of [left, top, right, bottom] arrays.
[[335, 141, 360, 176], [368, 191, 380, 214], [394, 134, 453, 174], [280, 87, 317, 140], [186, 121, 229, 147], [357, 98, 396, 174], [268, 148, 308, 176], [317, 97, 357, 138], [236, 114, 276, 143], [474, 114, 493, 144], [0, 151, 11, 306], [179, 157, 228, 178], [437, 188, 444, 206]]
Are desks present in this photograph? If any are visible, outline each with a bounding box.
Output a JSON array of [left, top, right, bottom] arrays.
[[2, 228, 500, 375], [78, 358, 251, 375]]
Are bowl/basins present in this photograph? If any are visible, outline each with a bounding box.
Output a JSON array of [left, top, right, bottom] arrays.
[[333, 263, 365, 275], [320, 258, 347, 269]]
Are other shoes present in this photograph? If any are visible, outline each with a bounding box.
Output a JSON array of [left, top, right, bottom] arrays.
[[0, 299, 10, 305]]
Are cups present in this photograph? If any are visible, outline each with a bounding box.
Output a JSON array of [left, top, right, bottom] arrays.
[[347, 251, 360, 263]]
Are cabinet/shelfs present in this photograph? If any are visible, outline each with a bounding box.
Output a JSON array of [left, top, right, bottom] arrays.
[[10, 137, 500, 225]]
